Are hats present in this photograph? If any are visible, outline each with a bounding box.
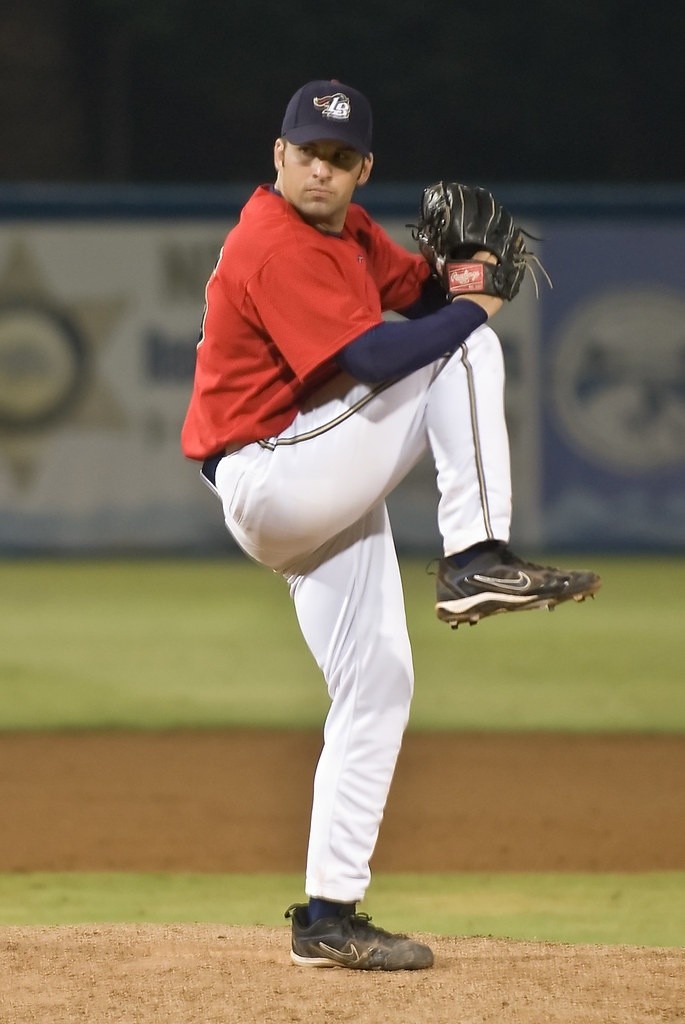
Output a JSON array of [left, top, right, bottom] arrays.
[[280, 80, 373, 154]]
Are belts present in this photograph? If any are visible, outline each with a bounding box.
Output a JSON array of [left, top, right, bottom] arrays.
[[203, 451, 220, 484]]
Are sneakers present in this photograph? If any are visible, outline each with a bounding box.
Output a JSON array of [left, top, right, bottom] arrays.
[[282, 902, 434, 970], [434, 541, 602, 629]]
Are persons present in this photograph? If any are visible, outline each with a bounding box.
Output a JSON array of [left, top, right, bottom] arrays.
[[182, 82, 603, 969]]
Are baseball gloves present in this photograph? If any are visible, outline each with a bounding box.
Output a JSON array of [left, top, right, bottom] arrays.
[[407, 178, 555, 301]]
